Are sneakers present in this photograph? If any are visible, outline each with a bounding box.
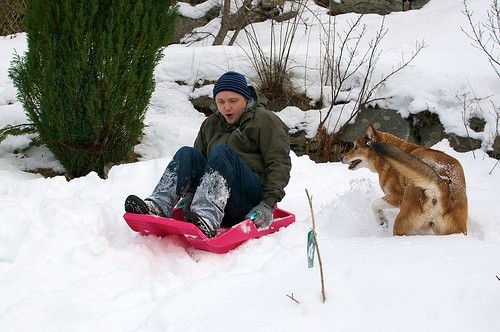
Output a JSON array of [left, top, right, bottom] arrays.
[[182, 209, 217, 238], [125, 195, 163, 216]]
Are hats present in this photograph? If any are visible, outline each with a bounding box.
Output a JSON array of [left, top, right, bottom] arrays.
[[213, 72, 252, 99]]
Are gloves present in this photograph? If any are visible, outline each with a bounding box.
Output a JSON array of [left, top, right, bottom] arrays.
[[177, 193, 192, 212], [245, 200, 274, 227]]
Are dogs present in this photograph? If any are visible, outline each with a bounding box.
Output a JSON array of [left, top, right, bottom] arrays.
[[336, 122, 469, 237]]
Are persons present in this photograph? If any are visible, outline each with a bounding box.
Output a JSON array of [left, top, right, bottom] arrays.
[[125, 71, 292, 238]]
